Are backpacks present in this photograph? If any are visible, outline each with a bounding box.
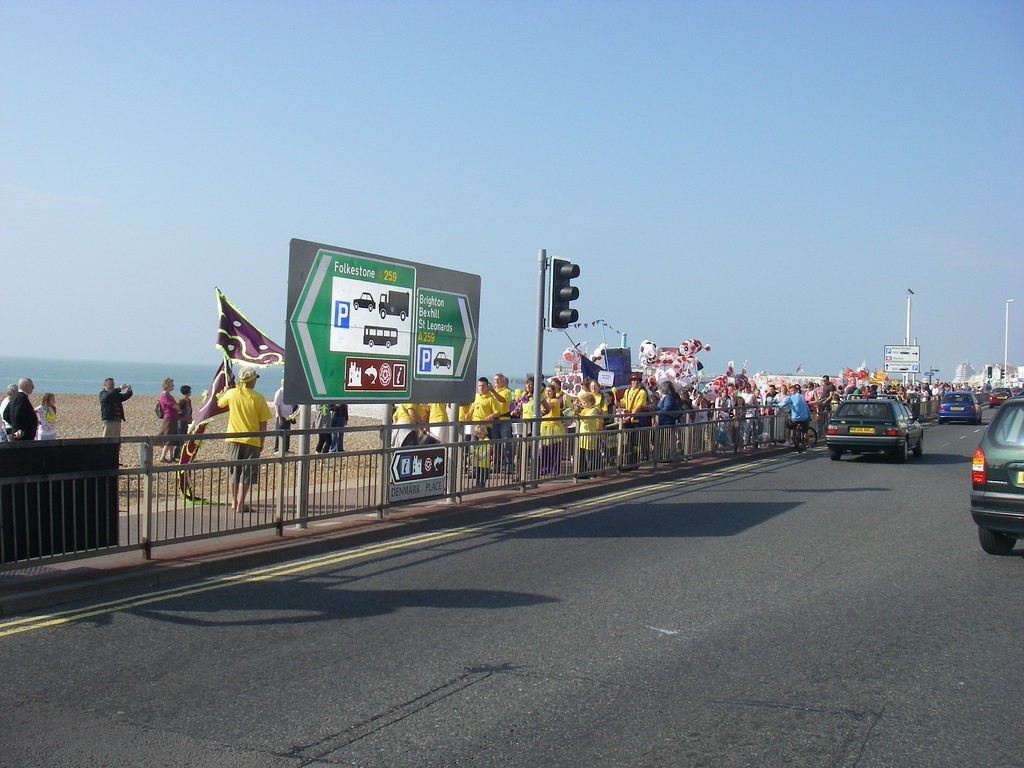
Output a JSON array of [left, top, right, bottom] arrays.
[[155, 402, 164, 419]]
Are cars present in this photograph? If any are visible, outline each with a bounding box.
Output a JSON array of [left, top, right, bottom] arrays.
[[989, 388, 1014, 408], [1012, 388, 1024, 396], [937, 391, 982, 425], [823, 398, 925, 461]]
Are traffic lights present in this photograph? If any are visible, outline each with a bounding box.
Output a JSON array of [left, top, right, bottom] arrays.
[[1002, 369, 1005, 378], [988, 367, 992, 378], [549, 257, 580, 330]]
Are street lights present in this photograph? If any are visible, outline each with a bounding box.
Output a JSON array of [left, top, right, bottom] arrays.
[[1004, 299, 1013, 387]]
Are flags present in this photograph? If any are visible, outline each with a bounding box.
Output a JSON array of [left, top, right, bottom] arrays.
[[216, 288, 286, 369], [175, 358, 239, 503]]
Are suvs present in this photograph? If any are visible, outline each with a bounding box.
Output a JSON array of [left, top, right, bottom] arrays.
[[969, 397, 1024, 555]]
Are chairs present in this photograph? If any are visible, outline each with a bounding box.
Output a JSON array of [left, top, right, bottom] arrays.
[[847, 409, 862, 417]]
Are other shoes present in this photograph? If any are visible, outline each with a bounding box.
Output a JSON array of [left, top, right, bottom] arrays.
[[159, 457, 178, 464], [782, 441, 791, 446], [512, 475, 521, 483]]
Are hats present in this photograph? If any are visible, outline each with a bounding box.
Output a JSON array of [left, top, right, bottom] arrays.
[[629, 373, 643, 383], [240, 367, 259, 383]]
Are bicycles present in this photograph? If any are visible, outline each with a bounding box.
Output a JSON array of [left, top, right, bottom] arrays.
[[792, 422, 818, 454]]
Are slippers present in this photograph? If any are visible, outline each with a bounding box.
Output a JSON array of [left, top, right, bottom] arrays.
[[231, 503, 255, 513]]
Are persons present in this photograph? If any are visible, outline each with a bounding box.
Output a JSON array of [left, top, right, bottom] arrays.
[[218, 367, 273, 513], [172, 385, 193, 463], [99, 377, 132, 466], [0, 377, 39, 443], [34, 392, 57, 440], [272, 374, 1014, 488], [154, 377, 181, 464]]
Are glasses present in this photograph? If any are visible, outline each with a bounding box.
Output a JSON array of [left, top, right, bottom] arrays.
[[632, 378, 641, 382], [822, 379, 828, 382]]
